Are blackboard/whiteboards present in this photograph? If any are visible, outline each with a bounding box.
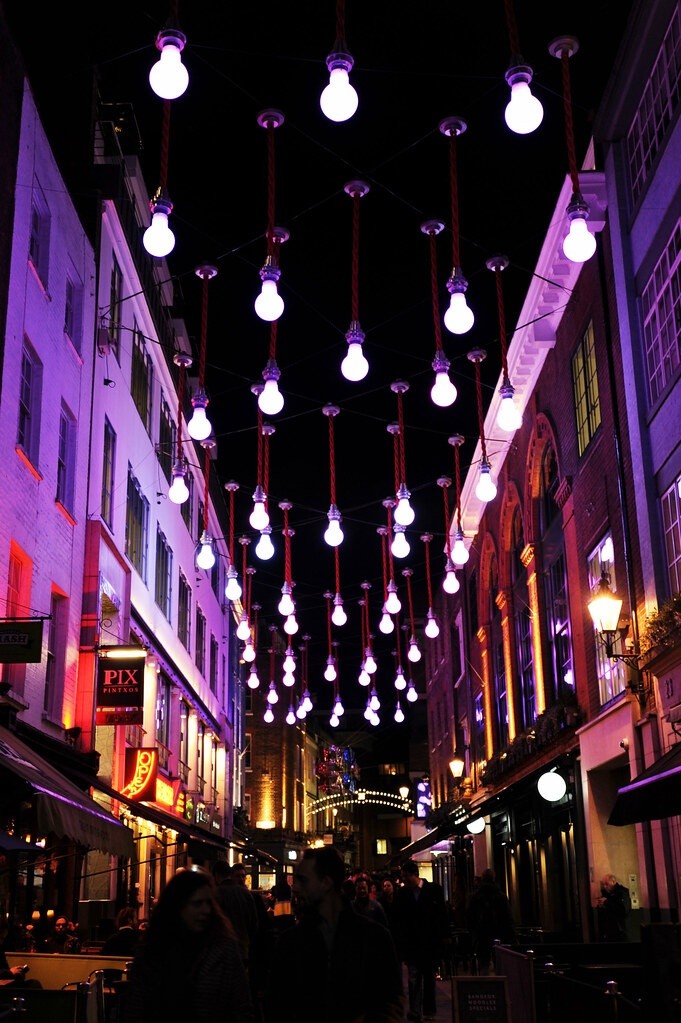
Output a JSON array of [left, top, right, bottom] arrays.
[[451, 976, 512, 1023]]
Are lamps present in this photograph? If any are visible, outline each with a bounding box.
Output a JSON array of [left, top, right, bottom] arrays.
[[449, 743, 471, 788], [587, 571, 653, 711], [65, 727, 81, 741]]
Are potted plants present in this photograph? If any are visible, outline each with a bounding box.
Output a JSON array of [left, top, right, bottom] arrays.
[[635, 593, 681, 671]]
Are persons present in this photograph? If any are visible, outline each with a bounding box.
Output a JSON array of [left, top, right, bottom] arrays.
[[101, 907, 143, 957], [39, 915, 79, 955], [212, 861, 256, 967], [341, 877, 397, 927], [0, 917, 42, 988], [117, 869, 253, 1023], [468, 868, 513, 961], [597, 875, 631, 942], [392, 863, 445, 1023], [256, 846, 407, 1023]]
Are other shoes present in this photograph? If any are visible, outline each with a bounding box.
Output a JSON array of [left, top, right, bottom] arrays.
[[423, 1013, 433, 1023], [406, 1011, 424, 1023]]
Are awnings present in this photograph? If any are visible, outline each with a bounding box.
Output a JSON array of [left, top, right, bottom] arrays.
[[0, 724, 133, 860], [607, 743, 681, 827]]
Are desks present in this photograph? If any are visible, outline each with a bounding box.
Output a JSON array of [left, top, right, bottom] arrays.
[[578, 963, 642, 969]]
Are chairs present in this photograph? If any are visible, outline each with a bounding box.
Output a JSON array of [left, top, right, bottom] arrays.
[[62, 967, 127, 993]]
